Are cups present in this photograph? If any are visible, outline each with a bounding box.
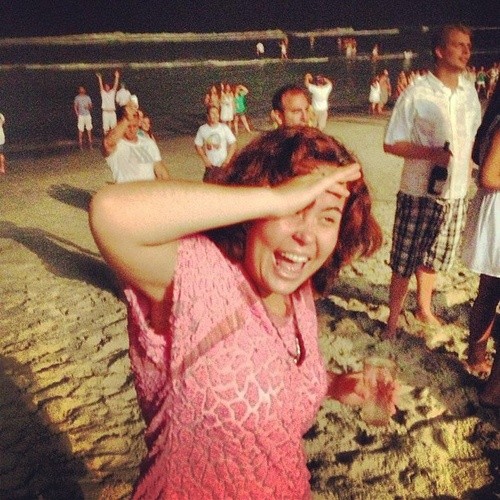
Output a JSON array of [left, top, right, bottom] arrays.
[[362, 357, 397, 426]]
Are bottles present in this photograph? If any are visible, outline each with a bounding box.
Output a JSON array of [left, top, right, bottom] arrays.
[[428, 141, 449, 194]]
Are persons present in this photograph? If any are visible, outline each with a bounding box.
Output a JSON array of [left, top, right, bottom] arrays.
[[139, 111, 156, 142], [383, 25, 483, 338], [194, 105, 237, 170], [304, 73, 332, 129], [460, 72, 500, 363], [0, 113, 6, 174], [88, 125, 398, 500], [369, 62, 500, 114], [96, 71, 119, 135], [204, 81, 252, 135], [271, 84, 309, 126], [116, 82, 132, 121], [102, 98, 171, 183], [257, 33, 415, 61], [74, 86, 96, 152]]
